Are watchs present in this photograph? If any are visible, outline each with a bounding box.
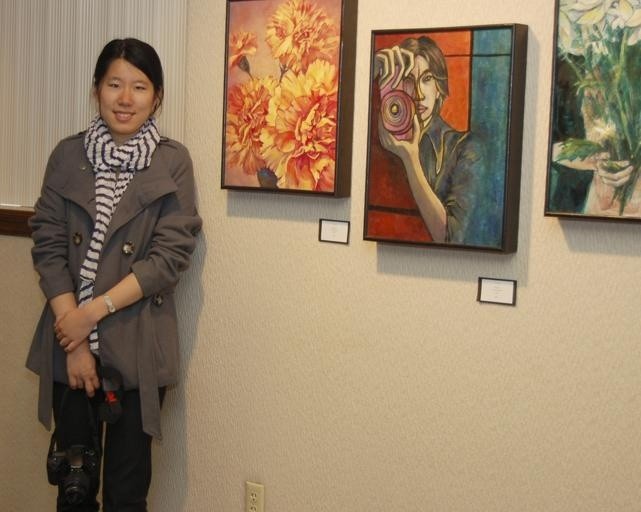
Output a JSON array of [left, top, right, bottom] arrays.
[[102, 292, 116, 315]]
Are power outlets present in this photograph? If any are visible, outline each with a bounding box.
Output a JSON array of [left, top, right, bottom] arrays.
[[244, 480, 265, 512]]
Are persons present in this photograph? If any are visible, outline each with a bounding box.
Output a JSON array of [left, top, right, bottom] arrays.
[[552, 143, 640, 219], [372, 36, 480, 245], [26, 38, 202, 512]]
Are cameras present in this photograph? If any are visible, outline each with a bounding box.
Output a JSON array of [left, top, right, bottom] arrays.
[[47, 444, 102, 504], [379, 64, 421, 141]]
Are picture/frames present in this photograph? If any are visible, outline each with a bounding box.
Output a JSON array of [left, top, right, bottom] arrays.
[[362, 23, 530, 254], [543, 0, 641, 223], [220, 1, 358, 199]]
